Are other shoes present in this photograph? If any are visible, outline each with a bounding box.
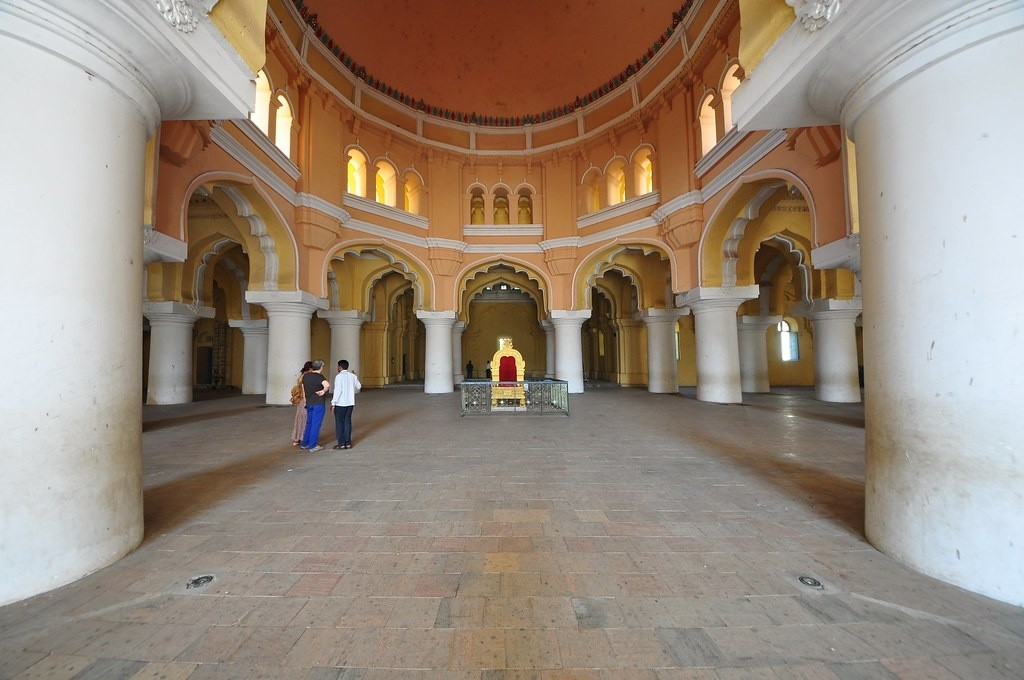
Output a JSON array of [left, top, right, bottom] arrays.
[[308, 445, 324, 452], [301, 445, 308, 449], [293, 442, 300, 446], [333, 445, 346, 450], [346, 444, 352, 449]]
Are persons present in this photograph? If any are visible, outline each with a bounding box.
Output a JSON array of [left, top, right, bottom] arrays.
[[331, 360, 361, 449], [466, 361, 473, 379], [292, 360, 330, 452], [486, 361, 491, 378]]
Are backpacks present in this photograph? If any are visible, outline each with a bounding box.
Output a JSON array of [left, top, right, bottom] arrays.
[[291, 377, 303, 404]]
